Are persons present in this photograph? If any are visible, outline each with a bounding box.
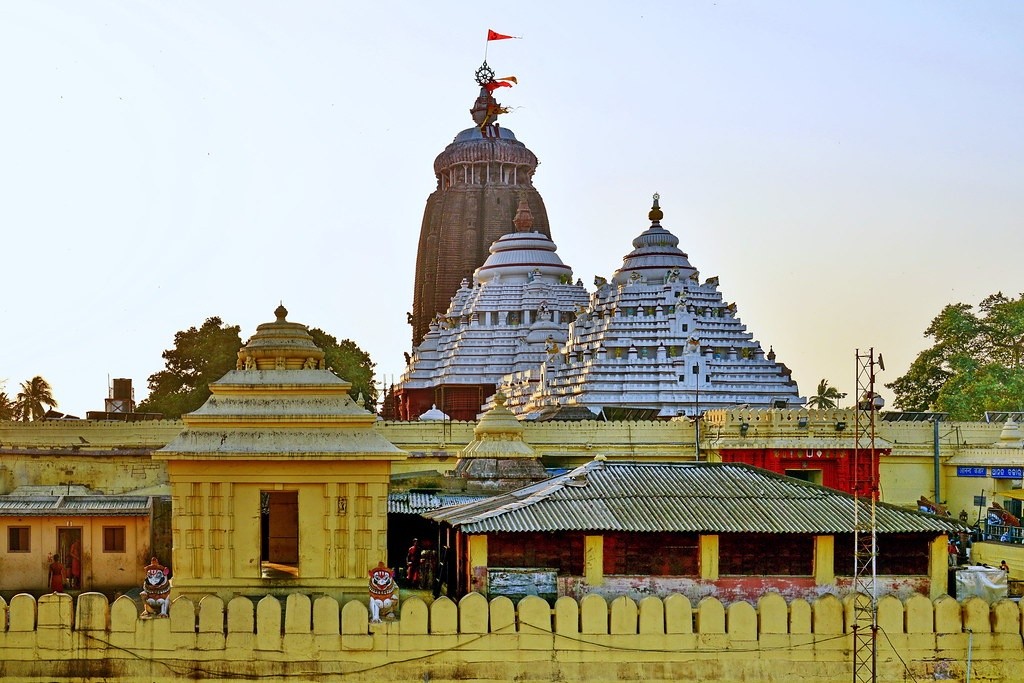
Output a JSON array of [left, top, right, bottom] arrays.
[[69, 538, 80, 589], [949, 541, 958, 565], [47, 553, 67, 594], [406, 538, 438, 590], [999, 560, 1009, 573]]
